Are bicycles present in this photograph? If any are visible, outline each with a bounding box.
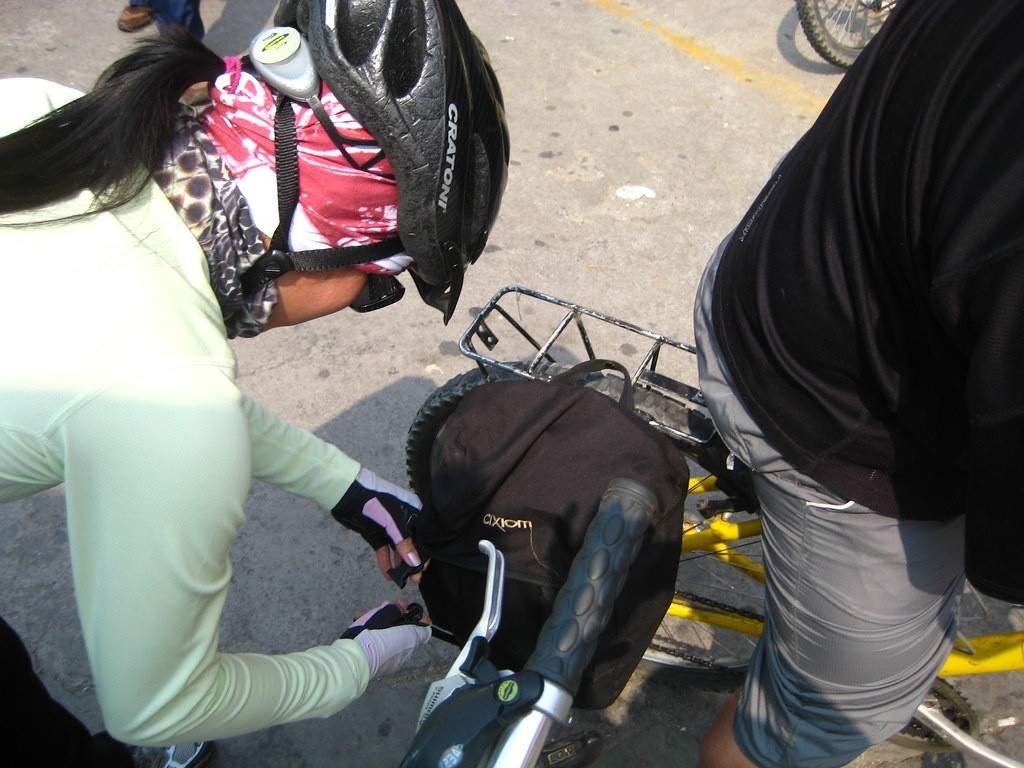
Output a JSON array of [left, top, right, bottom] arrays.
[[406, 285, 1024, 768], [796, 0, 898, 68], [398, 476, 659, 768]]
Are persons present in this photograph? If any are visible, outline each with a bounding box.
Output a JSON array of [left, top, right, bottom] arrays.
[[0, 2, 515, 768], [689, 1, 1024, 768]]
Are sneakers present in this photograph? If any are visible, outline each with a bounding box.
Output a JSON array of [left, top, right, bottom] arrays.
[[128, 739, 214, 768]]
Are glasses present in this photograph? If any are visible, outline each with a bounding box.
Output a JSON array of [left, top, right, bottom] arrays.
[[348, 271, 406, 313]]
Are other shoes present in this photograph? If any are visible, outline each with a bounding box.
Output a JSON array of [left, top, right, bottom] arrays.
[[185, 81, 212, 105], [118, 5, 156, 32]]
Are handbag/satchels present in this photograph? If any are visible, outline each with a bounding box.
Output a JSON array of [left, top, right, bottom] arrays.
[[386, 359, 691, 710]]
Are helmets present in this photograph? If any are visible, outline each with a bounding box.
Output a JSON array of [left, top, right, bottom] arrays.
[[262, 0, 511, 327]]
